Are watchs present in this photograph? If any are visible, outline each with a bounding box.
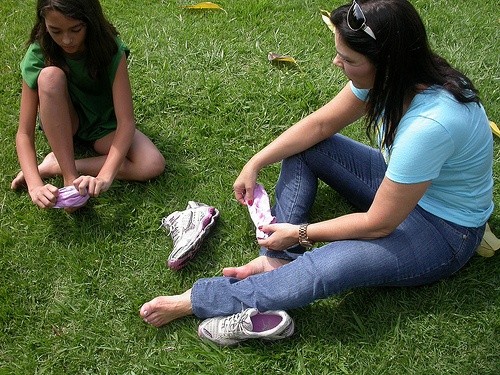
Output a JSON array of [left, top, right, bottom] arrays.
[[298, 223, 314, 248]]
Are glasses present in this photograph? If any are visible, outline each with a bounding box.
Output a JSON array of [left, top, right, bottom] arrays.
[[346, 0, 375, 40]]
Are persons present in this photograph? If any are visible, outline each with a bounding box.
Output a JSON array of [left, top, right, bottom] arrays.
[[9, 1, 165, 213], [140, 0, 495, 330]]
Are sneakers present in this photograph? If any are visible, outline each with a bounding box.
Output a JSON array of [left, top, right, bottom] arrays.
[[197, 307, 294, 346], [164, 201, 219, 270]]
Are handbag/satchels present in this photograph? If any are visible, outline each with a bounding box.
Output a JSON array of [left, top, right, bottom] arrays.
[[475, 222, 500, 259]]
